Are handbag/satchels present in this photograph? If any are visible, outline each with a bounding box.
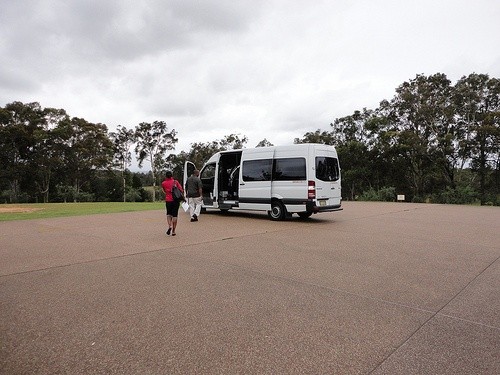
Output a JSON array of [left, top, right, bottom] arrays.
[[172, 179, 185, 203], [186, 193, 190, 198]]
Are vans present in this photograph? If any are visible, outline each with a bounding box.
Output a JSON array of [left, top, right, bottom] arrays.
[[182, 142, 344, 221]]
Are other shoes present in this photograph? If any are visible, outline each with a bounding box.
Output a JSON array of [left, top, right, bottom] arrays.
[[172, 233, 176, 236], [193, 214, 197, 220], [167, 227, 172, 235], [191, 219, 198, 222]]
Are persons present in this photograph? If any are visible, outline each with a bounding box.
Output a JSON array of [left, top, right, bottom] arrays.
[[184, 169, 204, 222], [161, 171, 185, 235]]
